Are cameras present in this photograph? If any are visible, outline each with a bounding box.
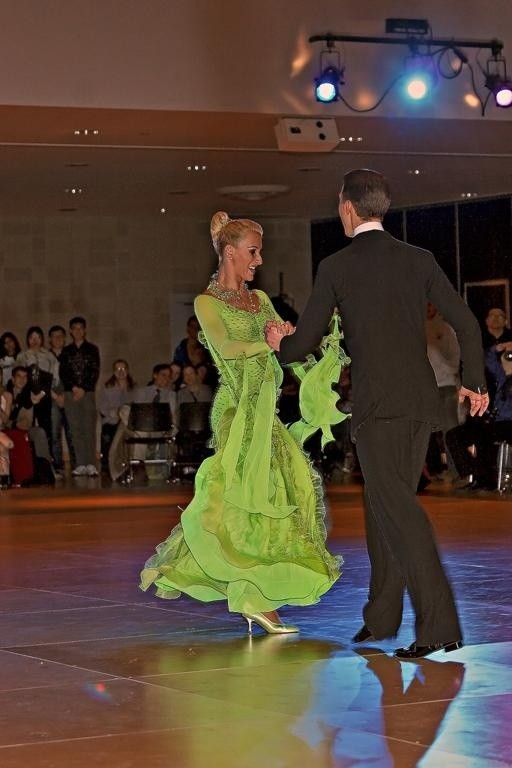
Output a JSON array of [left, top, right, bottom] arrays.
[[504, 350, 512, 362]]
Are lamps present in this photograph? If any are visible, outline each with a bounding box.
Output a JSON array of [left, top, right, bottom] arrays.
[[308, 16, 511, 116]]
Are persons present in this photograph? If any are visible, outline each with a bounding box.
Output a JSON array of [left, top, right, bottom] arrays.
[[424, 302, 512, 490], [139, 209, 355, 641], [281, 359, 363, 484], [1, 306, 221, 488], [264, 166, 491, 659]]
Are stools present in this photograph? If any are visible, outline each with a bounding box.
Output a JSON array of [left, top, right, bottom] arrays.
[[466, 442, 512, 494]]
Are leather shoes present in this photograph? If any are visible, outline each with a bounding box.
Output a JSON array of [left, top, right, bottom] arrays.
[[393, 640, 463, 661], [351, 624, 376, 644], [455, 479, 481, 494]]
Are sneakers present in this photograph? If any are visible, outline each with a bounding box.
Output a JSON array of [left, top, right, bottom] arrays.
[[70, 463, 86, 476], [86, 464, 99, 476]]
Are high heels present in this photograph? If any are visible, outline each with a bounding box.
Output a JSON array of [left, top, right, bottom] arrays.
[[241, 602, 300, 634]]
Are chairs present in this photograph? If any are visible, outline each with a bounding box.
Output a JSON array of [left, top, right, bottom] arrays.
[[120, 401, 177, 486], [173, 401, 216, 480]]
